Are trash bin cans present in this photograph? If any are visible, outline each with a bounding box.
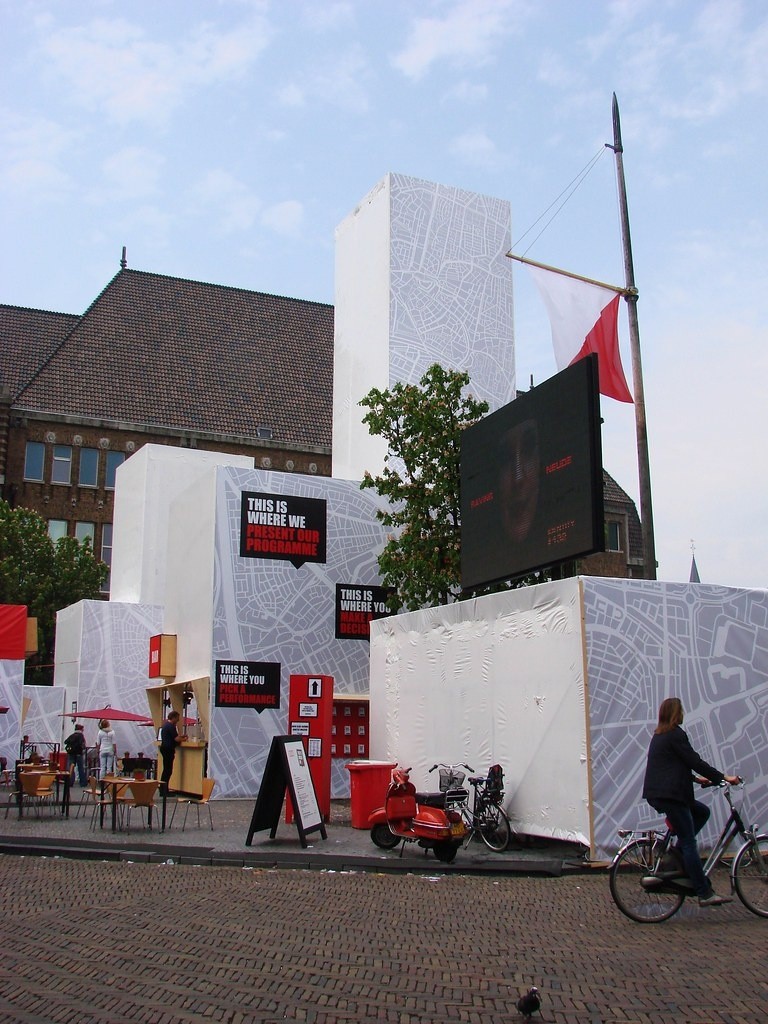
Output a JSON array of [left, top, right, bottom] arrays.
[[50, 752, 67, 770], [344, 760, 397, 830]]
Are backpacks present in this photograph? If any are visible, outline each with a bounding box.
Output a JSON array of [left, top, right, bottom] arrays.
[[487, 764, 505, 790]]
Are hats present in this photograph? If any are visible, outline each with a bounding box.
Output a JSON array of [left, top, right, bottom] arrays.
[[75, 724, 84, 730]]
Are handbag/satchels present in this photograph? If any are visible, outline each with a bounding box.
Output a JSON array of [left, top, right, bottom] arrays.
[[114, 755, 121, 778]]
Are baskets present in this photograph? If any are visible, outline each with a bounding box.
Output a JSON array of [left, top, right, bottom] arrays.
[[438, 769, 466, 792]]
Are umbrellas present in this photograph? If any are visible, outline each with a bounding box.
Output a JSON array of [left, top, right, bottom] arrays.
[[137, 716, 197, 727], [58, 705, 152, 722]]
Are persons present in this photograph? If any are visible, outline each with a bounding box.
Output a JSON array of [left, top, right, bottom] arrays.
[[64, 724, 88, 787], [159, 711, 188, 798], [642, 699, 740, 906], [96, 719, 117, 777]]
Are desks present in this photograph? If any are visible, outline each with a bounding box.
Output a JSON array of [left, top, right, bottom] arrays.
[[17, 770, 71, 820], [98, 778, 167, 834], [17, 763, 60, 772], [122, 758, 154, 780]]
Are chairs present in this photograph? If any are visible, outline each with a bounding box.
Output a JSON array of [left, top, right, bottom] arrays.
[[2, 759, 215, 836]]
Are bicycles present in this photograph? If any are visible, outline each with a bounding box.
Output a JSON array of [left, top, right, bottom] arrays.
[[606, 780, 768, 923], [428, 763, 512, 850]]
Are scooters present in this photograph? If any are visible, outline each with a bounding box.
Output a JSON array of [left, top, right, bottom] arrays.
[[367, 767, 468, 861]]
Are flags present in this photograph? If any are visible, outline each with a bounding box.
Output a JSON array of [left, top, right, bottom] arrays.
[[529, 265, 635, 404]]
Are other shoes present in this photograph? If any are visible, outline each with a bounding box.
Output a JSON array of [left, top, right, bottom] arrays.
[[699, 895, 734, 906], [159, 791, 175, 797]]
